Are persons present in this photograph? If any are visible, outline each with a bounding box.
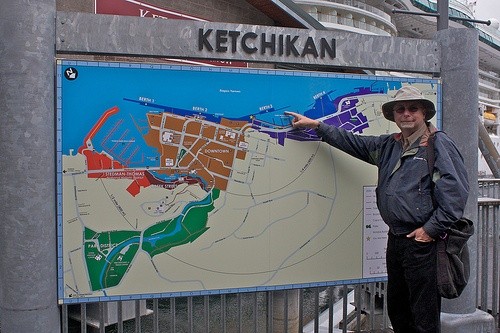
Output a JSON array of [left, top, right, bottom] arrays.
[[285, 86, 470, 333]]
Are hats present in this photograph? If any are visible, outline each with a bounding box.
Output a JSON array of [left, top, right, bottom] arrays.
[[381, 86, 436, 123]]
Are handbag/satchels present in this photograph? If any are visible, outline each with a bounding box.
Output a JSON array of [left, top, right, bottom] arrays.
[[435, 216, 475, 300]]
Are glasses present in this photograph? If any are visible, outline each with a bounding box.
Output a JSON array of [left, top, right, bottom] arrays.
[[389, 105, 428, 114]]
